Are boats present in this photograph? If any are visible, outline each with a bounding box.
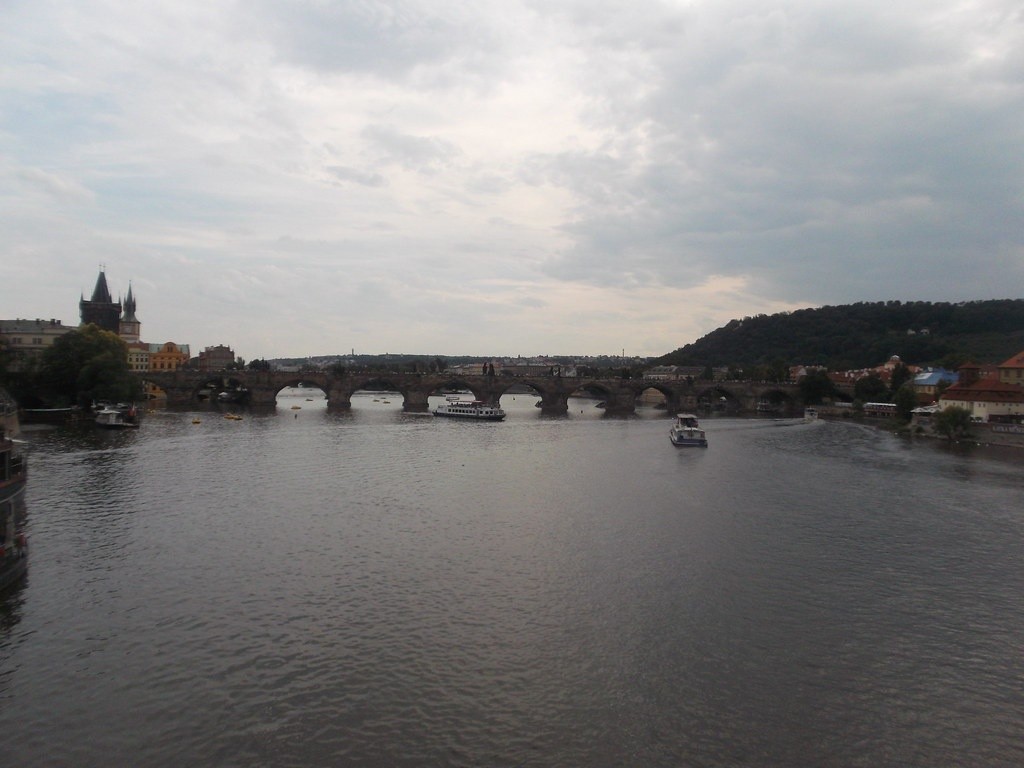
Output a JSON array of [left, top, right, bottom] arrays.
[[669, 413, 707, 447], [432, 400, 506, 419], [94, 409, 139, 429]]
